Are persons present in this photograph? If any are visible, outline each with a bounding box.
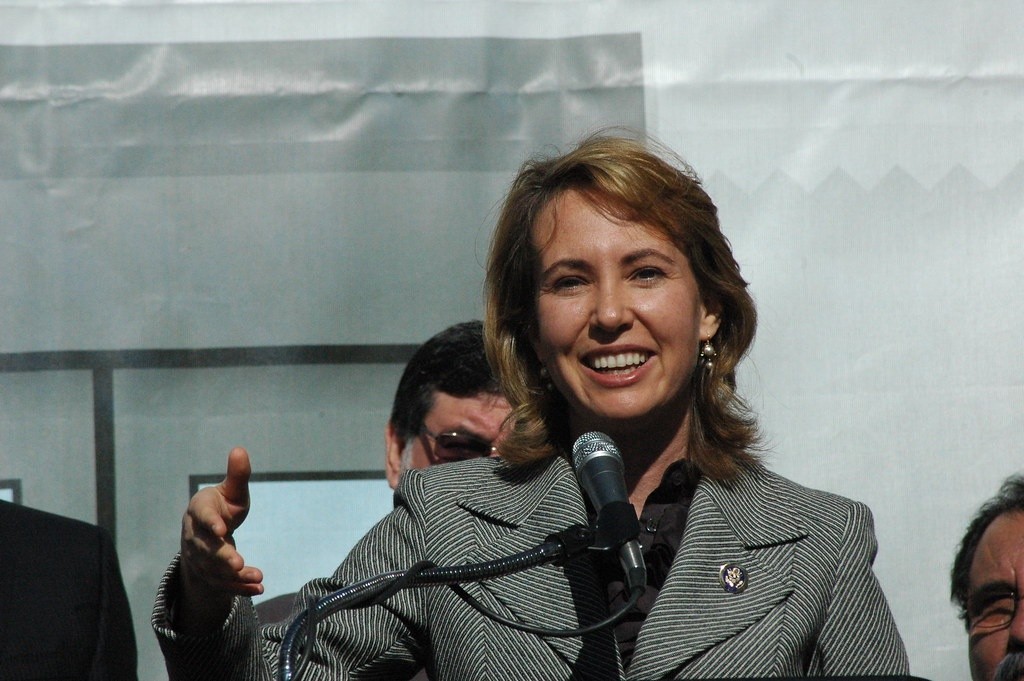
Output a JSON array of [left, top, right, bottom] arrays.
[[0, 499, 138, 681], [152, 134, 1024, 681]]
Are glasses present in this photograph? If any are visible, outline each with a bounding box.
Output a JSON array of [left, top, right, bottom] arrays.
[[957, 590, 1021, 631], [420, 423, 490, 461]]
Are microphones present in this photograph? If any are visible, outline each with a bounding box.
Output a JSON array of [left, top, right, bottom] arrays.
[[573, 431, 648, 594]]
